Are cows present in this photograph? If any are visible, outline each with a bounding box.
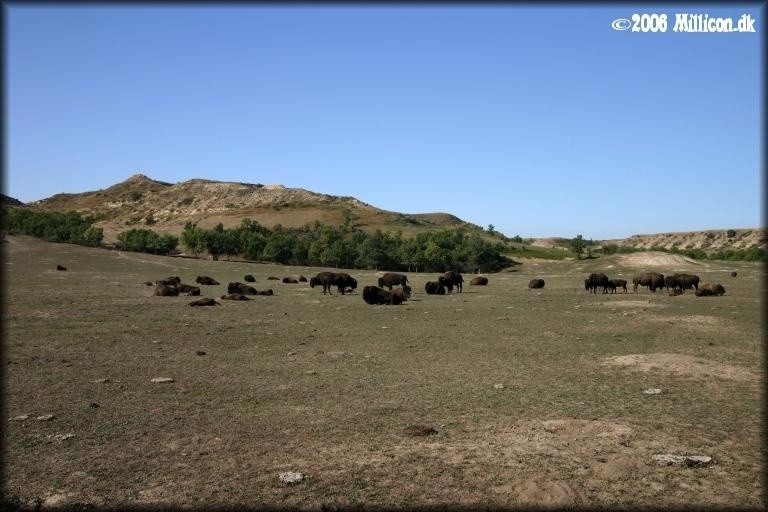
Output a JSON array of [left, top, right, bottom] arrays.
[[362, 272, 412, 305], [583, 272, 628, 294], [153, 271, 357, 307], [632, 272, 726, 297], [469, 276, 488, 286], [425, 271, 464, 295], [528, 278, 545, 289]]
